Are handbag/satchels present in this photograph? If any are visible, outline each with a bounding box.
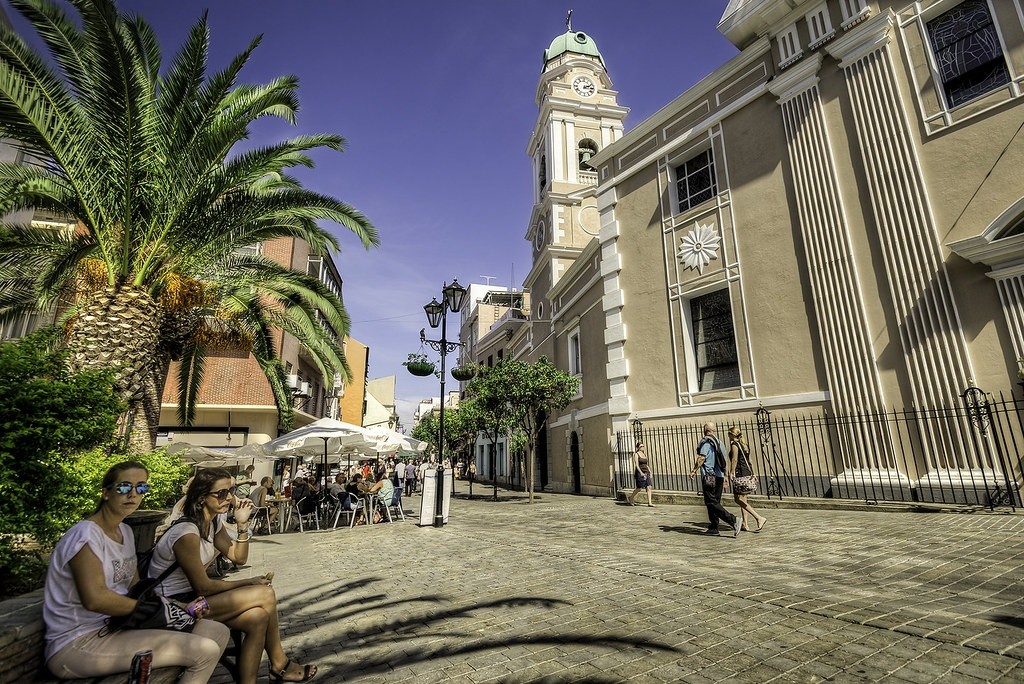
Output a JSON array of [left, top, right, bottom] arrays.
[[733, 476, 754, 493], [135, 549, 154, 580], [98, 578, 196, 637]]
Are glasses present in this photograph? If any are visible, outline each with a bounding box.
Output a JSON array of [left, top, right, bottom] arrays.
[[204, 486, 236, 499], [107, 481, 150, 495], [640, 445, 644, 448]]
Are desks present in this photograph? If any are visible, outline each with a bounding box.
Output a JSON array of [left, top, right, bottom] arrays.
[[264, 497, 293, 534]]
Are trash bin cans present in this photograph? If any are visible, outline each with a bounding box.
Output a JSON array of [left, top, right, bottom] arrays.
[[122, 511, 169, 552]]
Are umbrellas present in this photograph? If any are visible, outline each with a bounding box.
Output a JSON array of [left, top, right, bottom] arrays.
[[167, 414, 427, 498]]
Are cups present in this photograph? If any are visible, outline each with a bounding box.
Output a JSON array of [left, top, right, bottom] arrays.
[[275, 492, 281, 499]]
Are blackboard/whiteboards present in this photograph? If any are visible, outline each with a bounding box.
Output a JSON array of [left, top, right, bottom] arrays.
[[389, 471, 399, 487]]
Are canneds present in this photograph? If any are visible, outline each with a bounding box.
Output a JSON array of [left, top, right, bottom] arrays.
[[127, 650, 153, 684]]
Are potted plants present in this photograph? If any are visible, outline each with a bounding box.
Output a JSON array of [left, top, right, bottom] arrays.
[[402, 353, 442, 379], [450, 357, 486, 380]]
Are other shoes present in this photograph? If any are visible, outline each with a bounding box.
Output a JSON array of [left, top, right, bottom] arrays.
[[648, 504, 655, 507], [355, 519, 365, 525], [741, 527, 749, 531], [733, 516, 743, 536], [294, 523, 300, 531], [705, 529, 720, 536], [257, 528, 272, 535], [627, 497, 634, 506], [757, 517, 767, 531]]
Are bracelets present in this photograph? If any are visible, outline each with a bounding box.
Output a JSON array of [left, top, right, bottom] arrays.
[[234, 527, 250, 542]]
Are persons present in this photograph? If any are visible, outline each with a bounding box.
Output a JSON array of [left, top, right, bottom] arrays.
[[283, 457, 439, 522], [728, 427, 766, 530], [172, 476, 194, 520], [628, 443, 654, 507], [689, 423, 743, 536], [454, 458, 476, 479], [148, 468, 318, 684], [42, 460, 230, 684], [235, 465, 278, 535]]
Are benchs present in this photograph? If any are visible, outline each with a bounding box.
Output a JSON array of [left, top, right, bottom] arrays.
[[30, 633, 242, 684]]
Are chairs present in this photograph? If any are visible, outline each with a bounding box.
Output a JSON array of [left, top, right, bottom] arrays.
[[248, 483, 406, 535]]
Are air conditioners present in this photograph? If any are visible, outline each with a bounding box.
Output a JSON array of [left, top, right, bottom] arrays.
[[282, 375, 313, 398]]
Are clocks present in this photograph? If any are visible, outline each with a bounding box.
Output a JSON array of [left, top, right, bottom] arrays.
[[571, 74, 597, 98]]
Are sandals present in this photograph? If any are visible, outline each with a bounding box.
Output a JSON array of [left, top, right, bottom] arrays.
[[269, 659, 318, 684]]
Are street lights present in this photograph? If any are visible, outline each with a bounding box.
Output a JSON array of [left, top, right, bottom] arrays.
[[421, 275, 468, 529]]
